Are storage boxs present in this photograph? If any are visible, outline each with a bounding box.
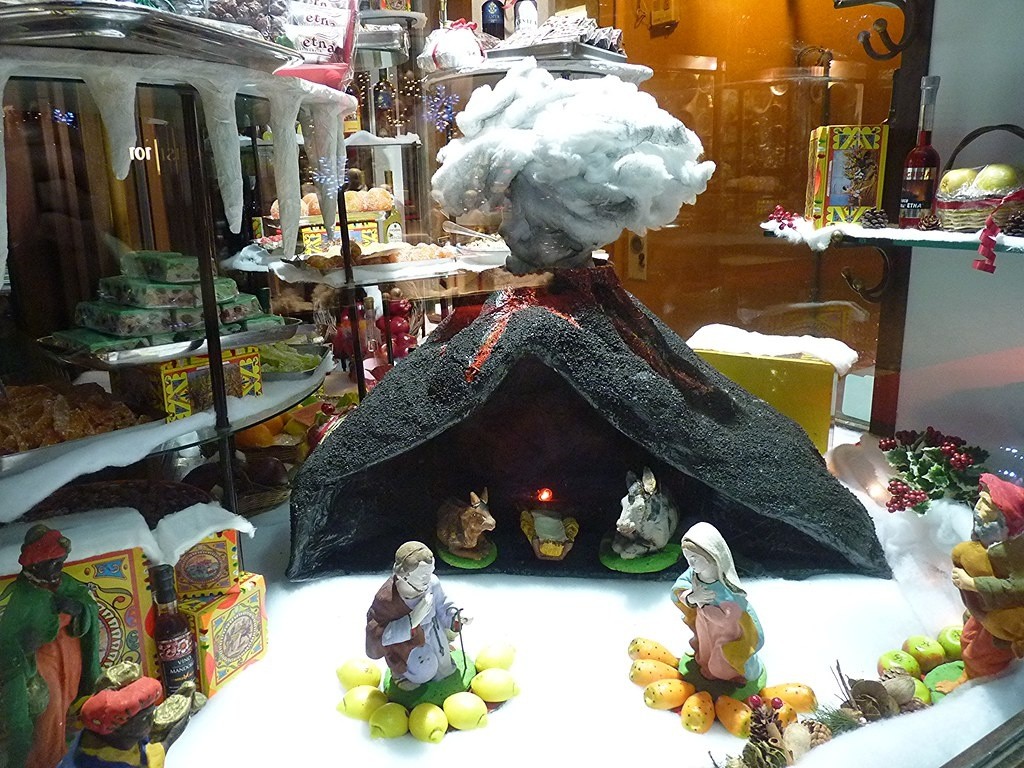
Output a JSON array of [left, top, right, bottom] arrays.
[[160, 502, 240, 597], [295, 219, 380, 255], [370, 0, 411, 12], [0, 505, 167, 705], [177, 570, 268, 699], [804, 124, 889, 230], [129, 345, 263, 424], [262, 209, 403, 244]]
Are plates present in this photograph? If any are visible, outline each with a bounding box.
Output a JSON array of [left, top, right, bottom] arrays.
[[454, 243, 515, 267]]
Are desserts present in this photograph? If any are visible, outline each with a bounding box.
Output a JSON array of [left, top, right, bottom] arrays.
[[51, 249, 285, 351]]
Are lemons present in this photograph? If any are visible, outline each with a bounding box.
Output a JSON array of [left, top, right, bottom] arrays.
[[237, 405, 308, 448]]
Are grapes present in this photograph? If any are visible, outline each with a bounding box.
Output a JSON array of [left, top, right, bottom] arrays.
[[877, 427, 975, 513], [768, 204, 799, 230]]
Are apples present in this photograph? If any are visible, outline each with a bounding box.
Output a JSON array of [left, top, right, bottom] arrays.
[[877, 624, 970, 704], [341, 297, 417, 381], [938, 162, 1024, 194]]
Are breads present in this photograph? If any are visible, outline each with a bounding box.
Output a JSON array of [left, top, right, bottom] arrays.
[[270, 186, 455, 269]]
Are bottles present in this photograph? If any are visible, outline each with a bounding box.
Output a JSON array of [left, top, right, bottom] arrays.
[[362, 296, 387, 392], [898, 77, 941, 229], [150, 564, 195, 699]]
